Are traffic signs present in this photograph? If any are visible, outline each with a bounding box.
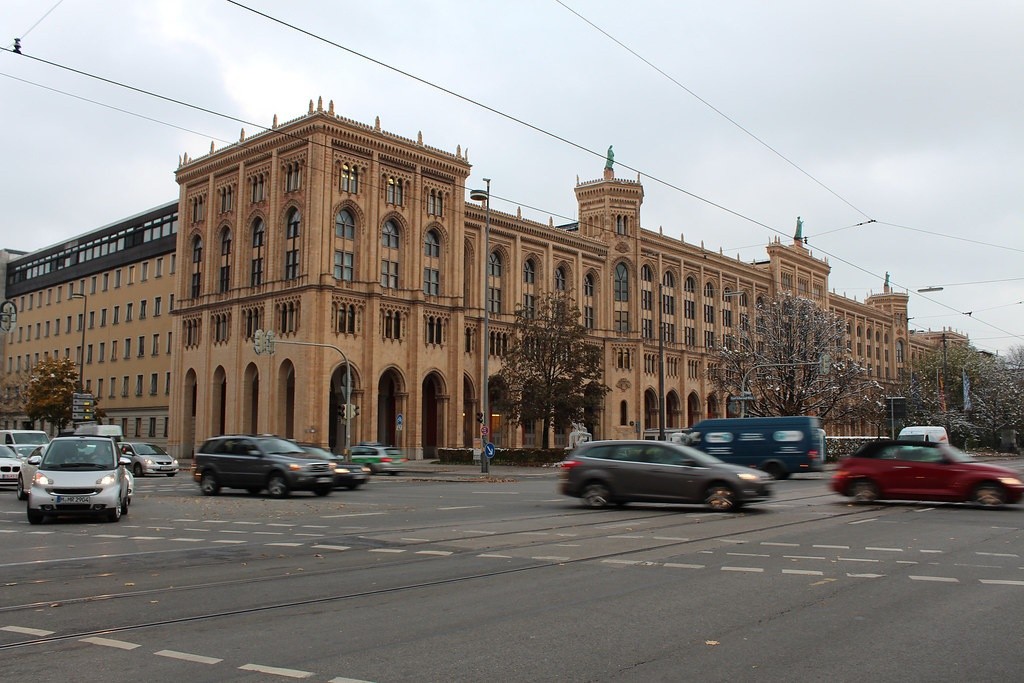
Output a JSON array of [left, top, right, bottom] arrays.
[[73, 393, 94, 421]]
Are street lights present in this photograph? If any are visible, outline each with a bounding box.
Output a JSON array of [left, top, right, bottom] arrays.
[[70, 293, 87, 394], [886, 396, 906, 440], [470, 178, 492, 473]]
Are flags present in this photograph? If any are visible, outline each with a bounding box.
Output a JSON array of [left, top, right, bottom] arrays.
[[912, 372, 920, 410], [938, 374, 946, 412], [962, 368, 972, 417]]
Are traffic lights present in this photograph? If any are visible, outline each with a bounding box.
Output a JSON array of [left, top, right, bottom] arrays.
[[477, 411, 484, 424], [351, 405, 360, 418], [338, 403, 346, 419]]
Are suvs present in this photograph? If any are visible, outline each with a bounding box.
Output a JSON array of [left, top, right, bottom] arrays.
[[190, 434, 337, 499], [558, 439, 775, 512]]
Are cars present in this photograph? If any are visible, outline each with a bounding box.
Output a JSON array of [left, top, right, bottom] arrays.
[[116, 442, 180, 477], [340, 443, 409, 472], [0, 446, 26, 482], [831, 439, 1024, 511], [16, 436, 135, 523], [292, 443, 371, 491]]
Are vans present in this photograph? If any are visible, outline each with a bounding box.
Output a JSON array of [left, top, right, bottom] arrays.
[[0, 429, 51, 459], [683, 416, 827, 480], [898, 425, 949, 446], [74, 424, 123, 450]]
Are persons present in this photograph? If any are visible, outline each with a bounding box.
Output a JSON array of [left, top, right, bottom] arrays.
[[604, 145, 614, 169], [91, 445, 109, 460], [794, 216, 804, 239], [885, 271, 890, 286]]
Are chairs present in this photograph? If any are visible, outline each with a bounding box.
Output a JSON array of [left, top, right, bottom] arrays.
[[57, 445, 79, 460]]
